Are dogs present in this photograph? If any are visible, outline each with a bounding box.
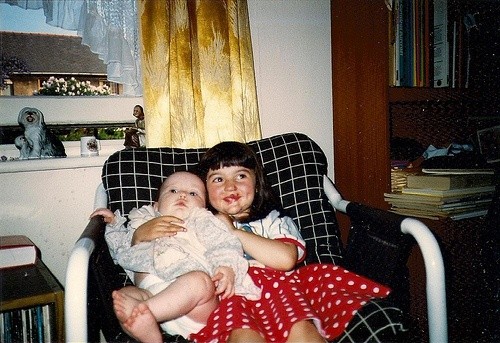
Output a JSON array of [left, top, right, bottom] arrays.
[[18, 107, 67, 159], [15, 136, 32, 160]]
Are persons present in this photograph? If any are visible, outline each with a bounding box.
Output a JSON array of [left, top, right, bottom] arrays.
[[129, 141, 326, 343], [91, 171, 250, 343]]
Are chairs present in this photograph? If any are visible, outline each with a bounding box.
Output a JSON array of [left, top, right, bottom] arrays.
[[62, 129, 450, 342]]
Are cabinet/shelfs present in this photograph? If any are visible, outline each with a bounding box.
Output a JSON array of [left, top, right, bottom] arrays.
[[329, 0, 499, 343], [1, 253, 66, 343]]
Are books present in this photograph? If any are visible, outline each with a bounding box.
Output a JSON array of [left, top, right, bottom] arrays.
[[387, 1, 499, 87], [0, 303, 59, 343], [0, 234, 38, 272], [384, 159, 496, 221]]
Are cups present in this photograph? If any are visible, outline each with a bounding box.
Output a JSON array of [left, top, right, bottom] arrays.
[[80, 135, 102, 158]]
